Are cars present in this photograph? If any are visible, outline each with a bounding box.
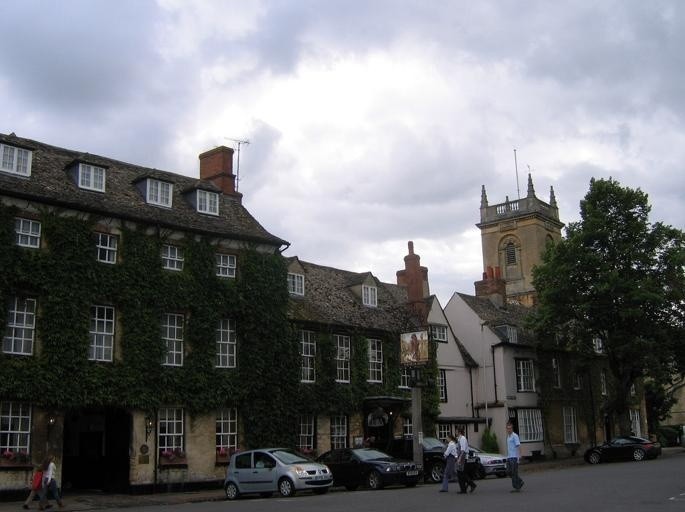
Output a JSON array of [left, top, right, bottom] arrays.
[[223, 448, 333, 499], [314, 448, 423, 489], [584, 437, 662, 464], [376, 437, 506, 483]]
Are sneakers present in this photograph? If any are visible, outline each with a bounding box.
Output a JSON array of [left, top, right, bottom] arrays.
[[510, 480, 524, 493], [23, 504, 30, 510], [45, 504, 53, 509], [456, 484, 476, 494], [440, 490, 448, 492]]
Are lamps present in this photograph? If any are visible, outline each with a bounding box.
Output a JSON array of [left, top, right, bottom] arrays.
[[144, 413, 155, 441], [44, 412, 59, 440]]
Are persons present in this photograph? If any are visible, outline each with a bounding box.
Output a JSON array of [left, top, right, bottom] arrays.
[[504, 422, 526, 493], [37, 454, 66, 510], [21, 464, 53, 508], [438, 433, 469, 492], [454, 428, 478, 494]]
